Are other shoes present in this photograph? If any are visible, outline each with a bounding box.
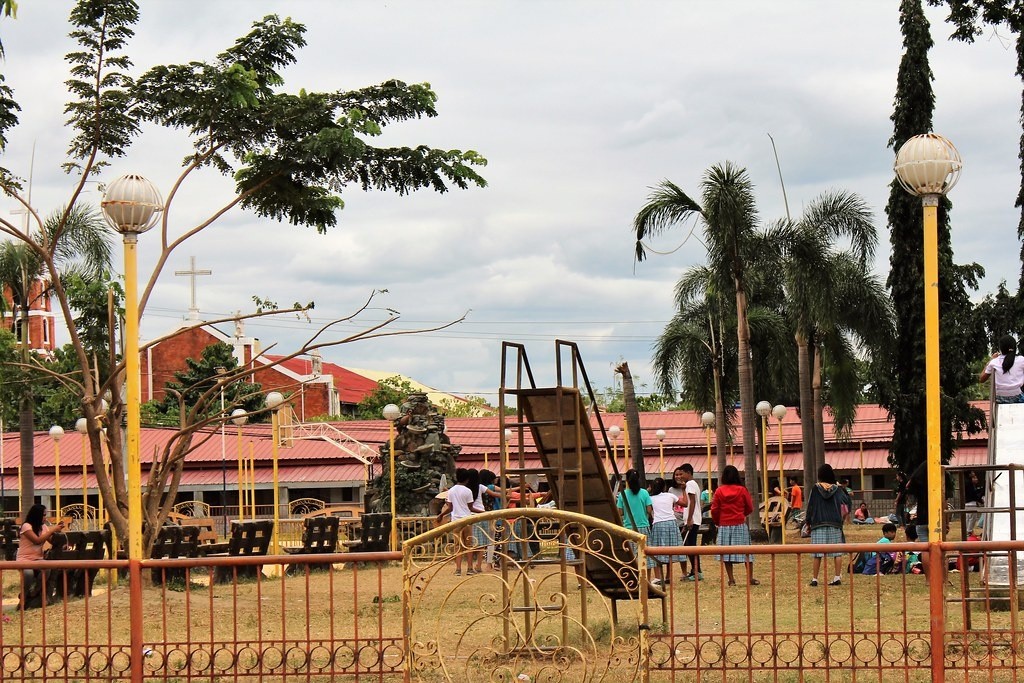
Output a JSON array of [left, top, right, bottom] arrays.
[[750, 579, 760, 585], [728, 581, 737, 586], [828, 580, 841, 586], [810, 581, 817, 587]]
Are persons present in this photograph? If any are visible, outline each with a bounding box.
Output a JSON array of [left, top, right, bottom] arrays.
[[701, 480, 709, 528], [906, 461, 954, 586], [805, 464, 849, 586], [668, 467, 687, 578], [855, 503, 870, 521], [617, 469, 660, 587], [979, 334, 1024, 403], [565, 534, 581, 589], [16, 504, 70, 611], [964, 470, 978, 536], [872, 524, 896, 557], [905, 525, 918, 542], [894, 471, 907, 527], [837, 479, 854, 525], [770, 479, 788, 511], [649, 478, 688, 584], [437, 468, 548, 576], [679, 463, 704, 580], [711, 465, 760, 586], [789, 475, 802, 528], [957, 533, 982, 571]]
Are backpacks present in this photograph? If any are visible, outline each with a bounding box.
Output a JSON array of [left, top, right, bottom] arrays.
[[862, 551, 892, 574], [848, 551, 870, 574]]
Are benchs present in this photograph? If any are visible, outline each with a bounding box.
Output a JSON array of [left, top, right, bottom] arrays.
[[0, 513, 394, 608]]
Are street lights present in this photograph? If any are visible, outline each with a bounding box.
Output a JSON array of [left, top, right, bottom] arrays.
[[702, 411, 715, 518], [231, 409, 249, 523], [893, 133, 962, 543], [656, 429, 666, 479], [772, 405, 787, 544], [602, 425, 620, 481], [756, 400, 772, 537], [101, 173, 164, 561], [49, 423, 66, 523], [265, 391, 285, 577], [381, 402, 400, 567], [75, 418, 88, 532], [505, 428, 513, 478]]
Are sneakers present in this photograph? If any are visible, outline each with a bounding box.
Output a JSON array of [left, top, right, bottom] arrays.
[[678, 574, 688, 581], [455, 569, 461, 576], [688, 571, 703, 580], [494, 563, 500, 571], [650, 578, 662, 585], [466, 568, 477, 575], [663, 577, 671, 584]]
